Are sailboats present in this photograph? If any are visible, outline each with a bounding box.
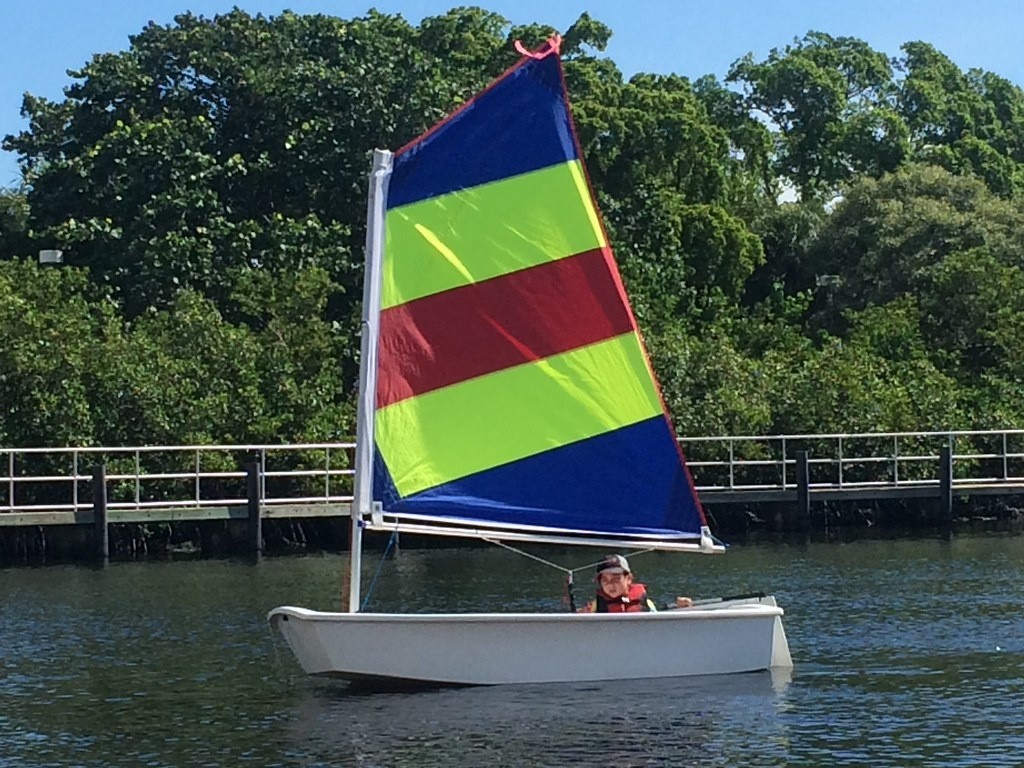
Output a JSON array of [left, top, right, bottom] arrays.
[[264, 32, 789, 688]]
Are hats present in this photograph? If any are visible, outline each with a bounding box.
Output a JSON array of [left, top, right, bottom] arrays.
[[595, 554, 630, 577]]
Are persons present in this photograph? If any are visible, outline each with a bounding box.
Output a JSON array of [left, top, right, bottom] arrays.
[[573, 553, 657, 614]]
